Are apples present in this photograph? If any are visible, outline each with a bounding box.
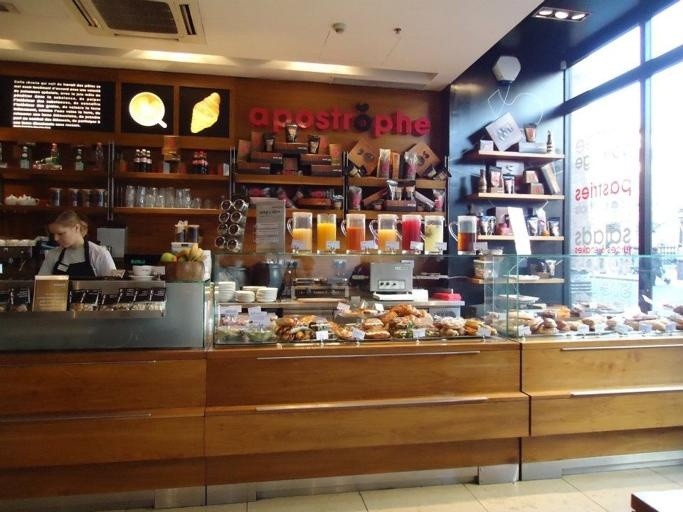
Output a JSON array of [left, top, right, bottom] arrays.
[[160, 253, 172, 262]]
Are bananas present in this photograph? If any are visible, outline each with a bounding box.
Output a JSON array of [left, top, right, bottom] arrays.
[[176, 243, 207, 262]]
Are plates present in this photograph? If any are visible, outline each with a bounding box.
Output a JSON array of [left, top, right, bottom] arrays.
[[127, 276, 155, 281], [215, 281, 278, 303]]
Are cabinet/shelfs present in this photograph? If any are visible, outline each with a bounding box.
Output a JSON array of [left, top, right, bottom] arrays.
[[0, 168, 109, 215], [112, 171, 233, 216], [204, 250, 529, 508], [462, 147, 566, 285], [484, 248, 681, 483], [344, 176, 448, 227], [232, 168, 345, 222]]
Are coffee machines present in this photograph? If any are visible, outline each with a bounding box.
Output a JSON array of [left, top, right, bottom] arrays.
[[0, 245, 44, 280]]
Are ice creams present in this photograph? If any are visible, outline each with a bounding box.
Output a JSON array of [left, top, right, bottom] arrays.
[[190, 92, 221, 134]]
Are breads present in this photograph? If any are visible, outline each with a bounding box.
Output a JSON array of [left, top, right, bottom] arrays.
[[488, 306, 683, 335], [275, 304, 479, 341]]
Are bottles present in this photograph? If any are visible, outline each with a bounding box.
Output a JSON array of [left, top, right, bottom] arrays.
[[218, 195, 226, 208], [48, 140, 59, 168], [547, 134, 553, 153], [20, 146, 30, 170], [478, 169, 486, 193], [133, 149, 152, 172], [74, 148, 84, 172], [94, 142, 105, 168], [191, 151, 208, 174]]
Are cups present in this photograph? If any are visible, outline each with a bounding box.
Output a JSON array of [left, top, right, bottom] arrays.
[[214, 199, 248, 254], [286, 211, 313, 255], [132, 265, 152, 276], [369, 213, 398, 254], [498, 223, 506, 236], [0, 239, 35, 245], [50, 187, 108, 206], [315, 213, 338, 256], [447, 215, 477, 256], [174, 223, 199, 243], [124, 184, 210, 209], [397, 214, 423, 254], [342, 213, 367, 254], [421, 215, 446, 255]]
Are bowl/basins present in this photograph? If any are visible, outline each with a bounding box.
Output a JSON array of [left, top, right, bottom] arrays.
[[164, 261, 204, 283], [246, 330, 271, 342], [215, 328, 243, 341]]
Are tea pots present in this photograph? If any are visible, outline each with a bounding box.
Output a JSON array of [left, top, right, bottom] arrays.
[[4, 193, 40, 206]]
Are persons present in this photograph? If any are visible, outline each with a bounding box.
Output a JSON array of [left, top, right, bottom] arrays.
[[37, 209, 117, 276], [652, 248, 671, 284]]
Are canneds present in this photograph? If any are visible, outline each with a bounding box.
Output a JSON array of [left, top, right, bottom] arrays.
[[48, 188, 108, 208]]
